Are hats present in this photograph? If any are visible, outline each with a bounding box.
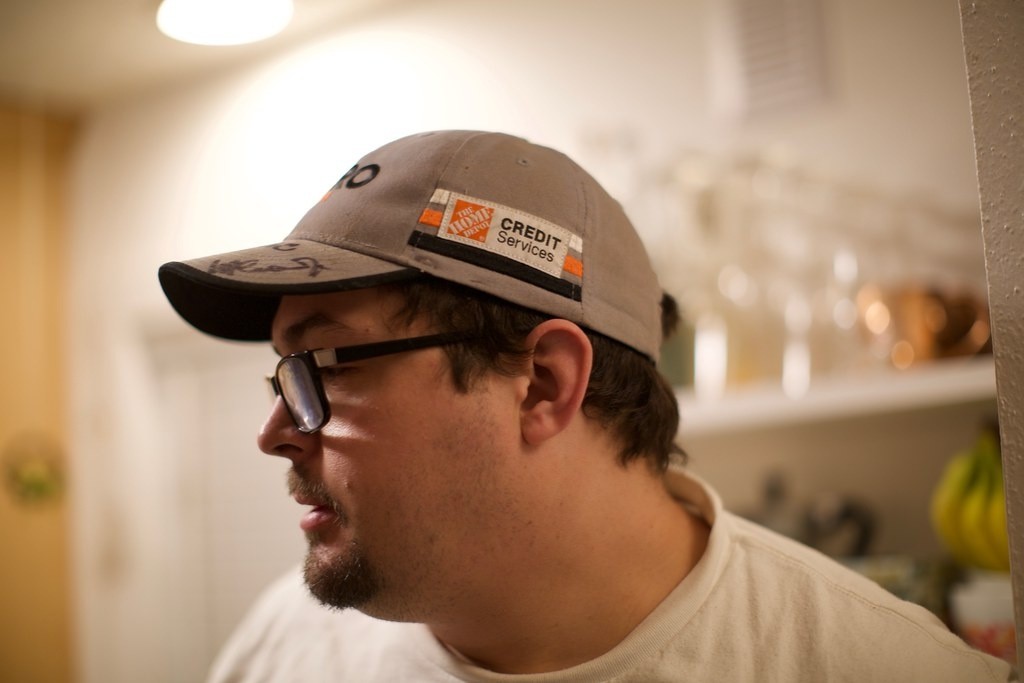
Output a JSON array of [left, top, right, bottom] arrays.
[[158, 130, 664, 368]]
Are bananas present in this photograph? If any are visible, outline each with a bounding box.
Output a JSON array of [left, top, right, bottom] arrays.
[[929, 421, 1012, 573]]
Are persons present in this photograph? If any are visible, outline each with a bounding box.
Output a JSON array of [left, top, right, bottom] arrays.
[[160, 128, 1019, 683]]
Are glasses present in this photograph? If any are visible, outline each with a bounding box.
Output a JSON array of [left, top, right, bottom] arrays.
[[265, 324, 532, 434]]
